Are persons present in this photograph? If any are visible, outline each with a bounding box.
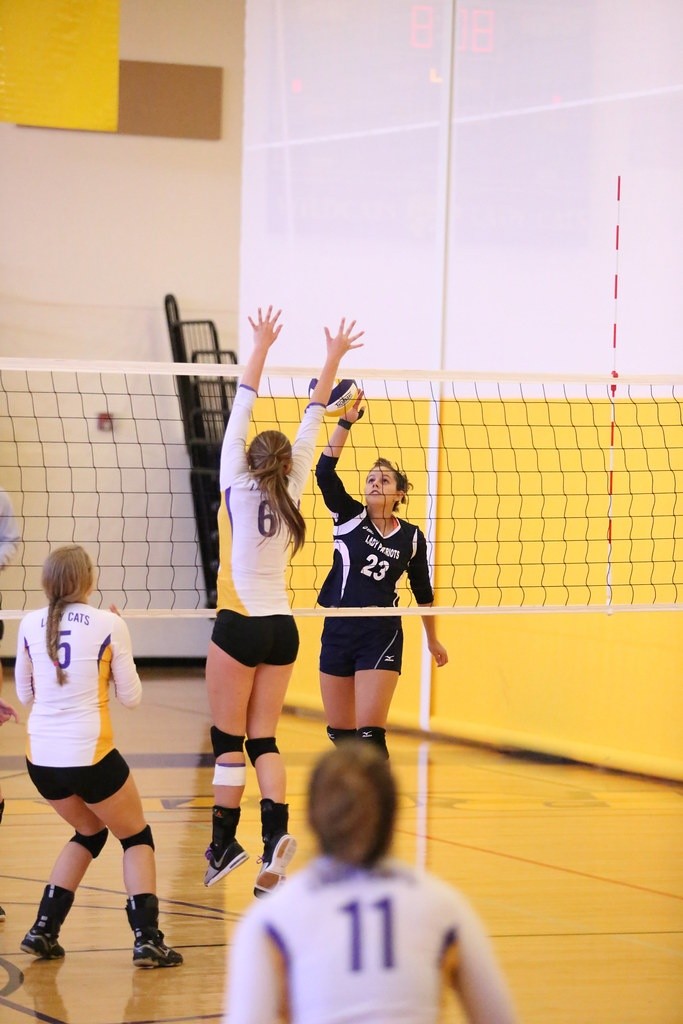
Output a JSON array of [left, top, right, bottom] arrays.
[[224, 737, 520, 1023], [14, 543, 190, 970], [1, 486, 22, 921], [314, 385, 451, 764], [203, 301, 368, 900]]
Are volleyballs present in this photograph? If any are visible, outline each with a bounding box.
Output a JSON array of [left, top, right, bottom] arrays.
[[309, 377, 358, 417]]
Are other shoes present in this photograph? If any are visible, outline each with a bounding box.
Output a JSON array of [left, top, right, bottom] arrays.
[[0, 905, 6, 923]]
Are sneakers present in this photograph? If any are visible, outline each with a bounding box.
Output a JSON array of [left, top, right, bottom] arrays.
[[253, 833, 298, 899], [20, 926, 64, 958], [133, 930, 183, 967], [204, 841, 250, 887]]
